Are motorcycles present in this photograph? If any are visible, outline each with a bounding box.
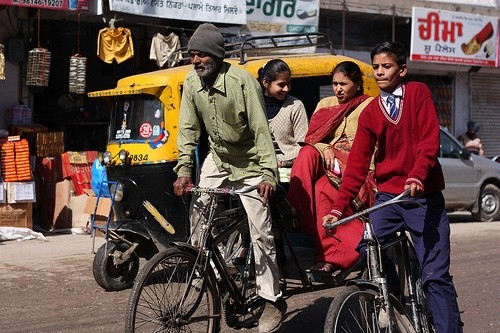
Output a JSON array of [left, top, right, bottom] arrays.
[[84, 31, 382, 291]]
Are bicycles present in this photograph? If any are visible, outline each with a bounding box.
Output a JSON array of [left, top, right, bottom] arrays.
[[322, 186, 438, 333]]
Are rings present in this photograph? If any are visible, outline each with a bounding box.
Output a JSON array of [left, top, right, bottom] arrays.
[[327, 160, 330, 164]]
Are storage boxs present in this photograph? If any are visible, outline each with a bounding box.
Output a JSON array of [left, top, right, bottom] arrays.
[[0, 104, 114, 237]]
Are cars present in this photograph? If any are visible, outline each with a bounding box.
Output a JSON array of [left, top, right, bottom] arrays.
[[438, 124, 500, 223]]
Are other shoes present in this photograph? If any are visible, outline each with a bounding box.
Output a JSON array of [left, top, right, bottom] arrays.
[[190, 278, 202, 289], [378, 303, 395, 328], [257, 296, 287, 333]]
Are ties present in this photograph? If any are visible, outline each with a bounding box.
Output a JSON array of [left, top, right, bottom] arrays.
[[387, 93, 399, 120]]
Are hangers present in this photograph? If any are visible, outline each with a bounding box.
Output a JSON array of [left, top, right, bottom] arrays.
[[160, 25, 172, 35], [107, 18, 119, 29]]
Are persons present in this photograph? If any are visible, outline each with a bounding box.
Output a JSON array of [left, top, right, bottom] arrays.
[[173, 24, 288, 333], [256, 59, 308, 183], [457, 121, 484, 157], [275, 61, 379, 273], [55, 91, 84, 113], [322, 41, 464, 333]]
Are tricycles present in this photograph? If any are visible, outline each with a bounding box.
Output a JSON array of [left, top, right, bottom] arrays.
[[124, 183, 328, 333]]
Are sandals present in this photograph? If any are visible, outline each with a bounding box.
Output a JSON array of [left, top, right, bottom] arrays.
[[309, 262, 336, 272], [281, 196, 298, 230]]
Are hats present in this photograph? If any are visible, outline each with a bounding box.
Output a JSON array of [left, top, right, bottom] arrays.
[[187, 22, 225, 61]]
